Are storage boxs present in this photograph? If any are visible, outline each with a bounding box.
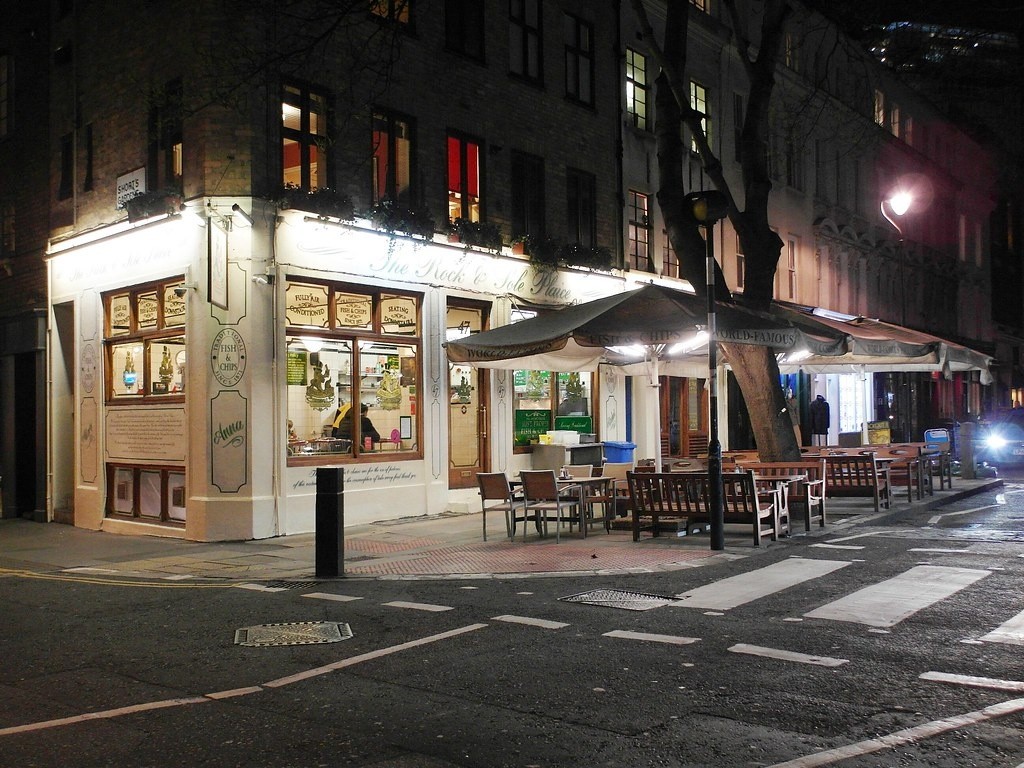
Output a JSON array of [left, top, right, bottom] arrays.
[[547, 430, 578, 443], [580, 433, 597, 443]]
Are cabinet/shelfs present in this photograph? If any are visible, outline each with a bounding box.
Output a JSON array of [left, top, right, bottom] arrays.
[[338, 371, 384, 392]]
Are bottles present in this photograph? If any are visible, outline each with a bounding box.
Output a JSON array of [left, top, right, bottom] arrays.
[[735, 464, 739, 473], [740, 465, 743, 473], [559, 466, 564, 476]]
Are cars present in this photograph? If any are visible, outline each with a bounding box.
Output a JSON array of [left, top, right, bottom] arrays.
[[977, 421, 1024, 470]]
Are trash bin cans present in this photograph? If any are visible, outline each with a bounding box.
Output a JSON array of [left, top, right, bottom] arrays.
[[861, 419, 891, 444], [602, 440, 638, 463]]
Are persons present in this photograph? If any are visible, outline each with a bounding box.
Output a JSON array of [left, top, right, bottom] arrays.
[[786, 388, 802, 449], [810, 395, 831, 434], [1010, 401, 1024, 428], [330, 402, 381, 453], [286, 419, 293, 440]]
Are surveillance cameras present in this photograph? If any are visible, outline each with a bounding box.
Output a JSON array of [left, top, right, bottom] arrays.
[[258, 278, 268, 284], [174, 288, 187, 297]]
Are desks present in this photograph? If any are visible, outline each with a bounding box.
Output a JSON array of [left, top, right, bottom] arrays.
[[875, 457, 903, 507], [507, 475, 616, 538], [686, 475, 806, 541], [532, 443, 604, 477], [372, 438, 402, 453]]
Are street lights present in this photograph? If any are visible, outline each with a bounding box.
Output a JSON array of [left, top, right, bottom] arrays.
[[683, 188, 729, 550]]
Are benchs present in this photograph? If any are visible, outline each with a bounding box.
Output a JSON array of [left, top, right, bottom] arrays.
[[561, 442, 951, 546]]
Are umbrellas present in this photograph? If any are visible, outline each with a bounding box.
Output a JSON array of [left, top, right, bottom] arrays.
[[440, 278, 992, 473]]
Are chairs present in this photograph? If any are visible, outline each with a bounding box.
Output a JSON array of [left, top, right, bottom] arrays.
[[474, 462, 635, 543]]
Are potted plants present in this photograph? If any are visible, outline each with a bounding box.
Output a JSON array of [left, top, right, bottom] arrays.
[[279, 180, 613, 273], [116, 170, 185, 222]]
